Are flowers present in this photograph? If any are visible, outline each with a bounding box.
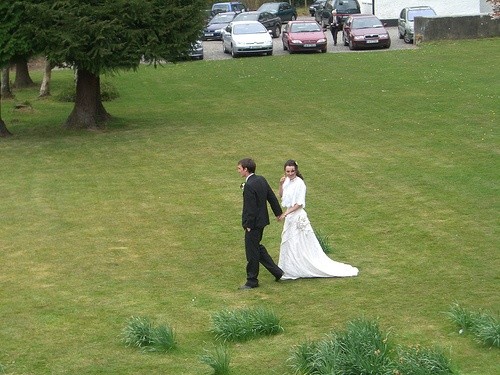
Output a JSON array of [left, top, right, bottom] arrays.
[[239, 182, 245, 191], [294, 161, 299, 166]]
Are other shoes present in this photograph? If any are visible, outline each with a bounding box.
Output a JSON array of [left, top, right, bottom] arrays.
[[238, 286, 251, 289], [275, 271, 284, 281]]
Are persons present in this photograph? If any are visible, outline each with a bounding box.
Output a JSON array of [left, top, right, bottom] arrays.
[[328, 9, 341, 46], [276, 159, 360, 279], [237, 158, 285, 290]]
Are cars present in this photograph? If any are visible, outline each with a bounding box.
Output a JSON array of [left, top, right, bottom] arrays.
[[282, 19, 328, 54], [200, 11, 242, 41], [254, 2, 298, 23], [231, 11, 282, 38], [173, 39, 205, 61], [397, 6, 439, 44], [342, 13, 391, 50], [309, 0, 327, 17], [220, 20, 274, 58]]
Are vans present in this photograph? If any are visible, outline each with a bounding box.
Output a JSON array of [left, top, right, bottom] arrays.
[[210, 2, 250, 18], [314, 0, 361, 31]]
